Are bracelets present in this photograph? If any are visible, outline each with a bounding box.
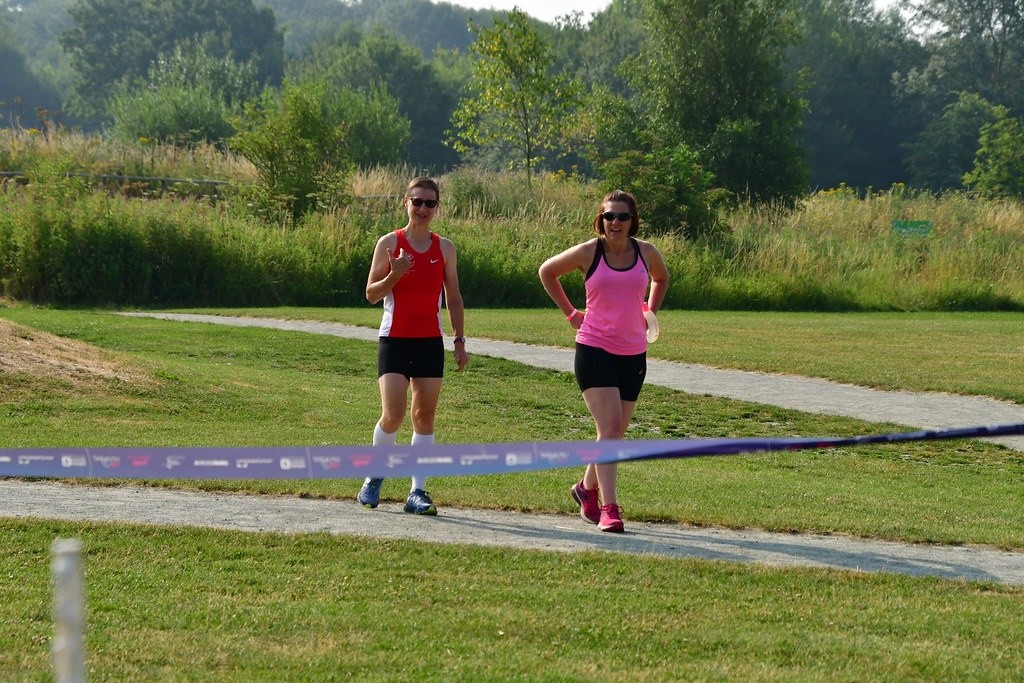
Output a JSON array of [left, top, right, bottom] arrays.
[[567, 309, 577, 322]]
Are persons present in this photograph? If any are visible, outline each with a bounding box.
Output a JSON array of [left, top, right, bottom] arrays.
[[357, 177, 471, 516], [538, 190, 669, 533]]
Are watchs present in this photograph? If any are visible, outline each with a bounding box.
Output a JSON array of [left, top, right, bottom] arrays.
[[453, 337, 465, 344]]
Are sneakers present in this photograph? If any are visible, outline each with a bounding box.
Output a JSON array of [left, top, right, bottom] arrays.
[[358, 476, 384, 508], [597, 503, 624, 532], [404, 489, 437, 515], [571, 479, 602, 524]]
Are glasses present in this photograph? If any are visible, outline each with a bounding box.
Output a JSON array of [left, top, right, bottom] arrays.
[[601, 211, 633, 221], [407, 196, 439, 209]]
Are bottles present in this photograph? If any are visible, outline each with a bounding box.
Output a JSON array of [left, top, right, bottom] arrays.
[[642, 301, 659, 343]]
